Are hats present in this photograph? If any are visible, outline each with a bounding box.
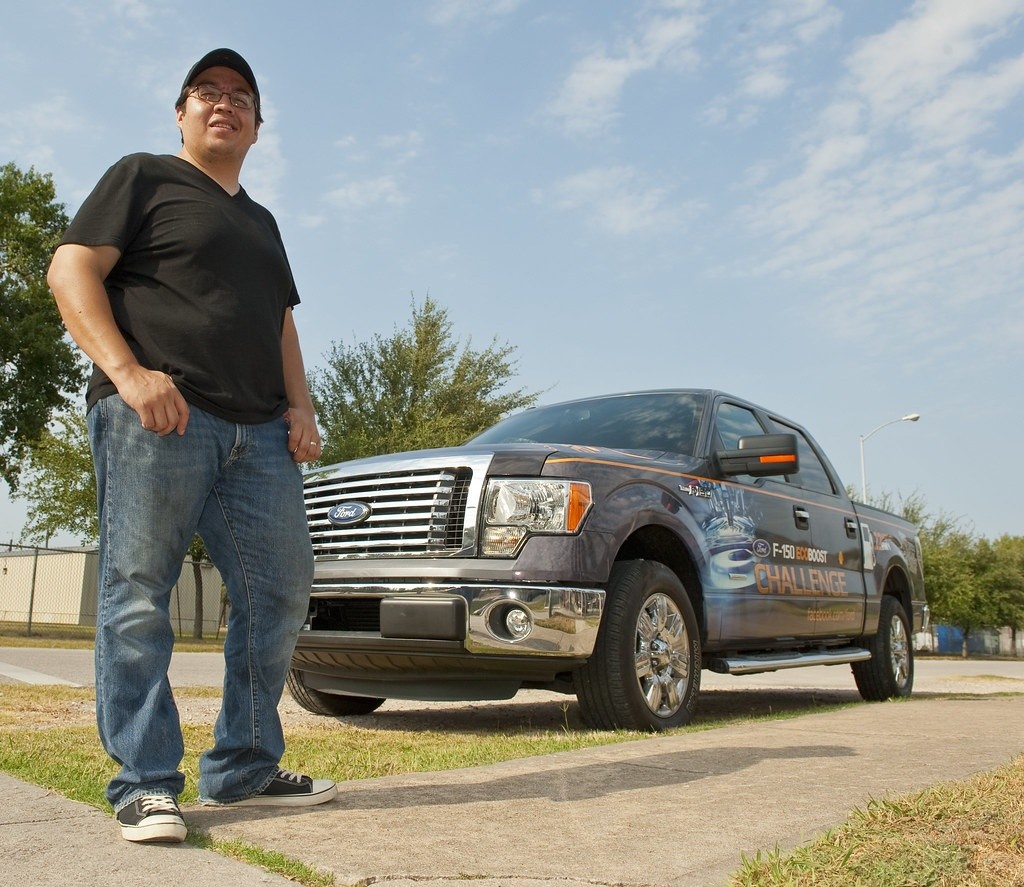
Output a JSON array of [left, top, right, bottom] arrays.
[[179, 48, 261, 112]]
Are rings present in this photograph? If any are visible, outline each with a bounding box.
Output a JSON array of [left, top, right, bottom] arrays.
[[310, 442, 316, 445]]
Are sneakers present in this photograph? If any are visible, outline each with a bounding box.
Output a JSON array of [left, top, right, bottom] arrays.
[[197, 766, 338, 807], [117, 793, 187, 843]]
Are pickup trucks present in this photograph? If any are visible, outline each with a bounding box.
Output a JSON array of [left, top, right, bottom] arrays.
[[283, 393, 934, 732]]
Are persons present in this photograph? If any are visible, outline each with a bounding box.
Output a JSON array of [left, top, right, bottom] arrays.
[[47, 48, 336, 842]]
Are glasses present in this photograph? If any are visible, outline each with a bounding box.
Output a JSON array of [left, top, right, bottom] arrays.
[[182, 86, 258, 115]]
